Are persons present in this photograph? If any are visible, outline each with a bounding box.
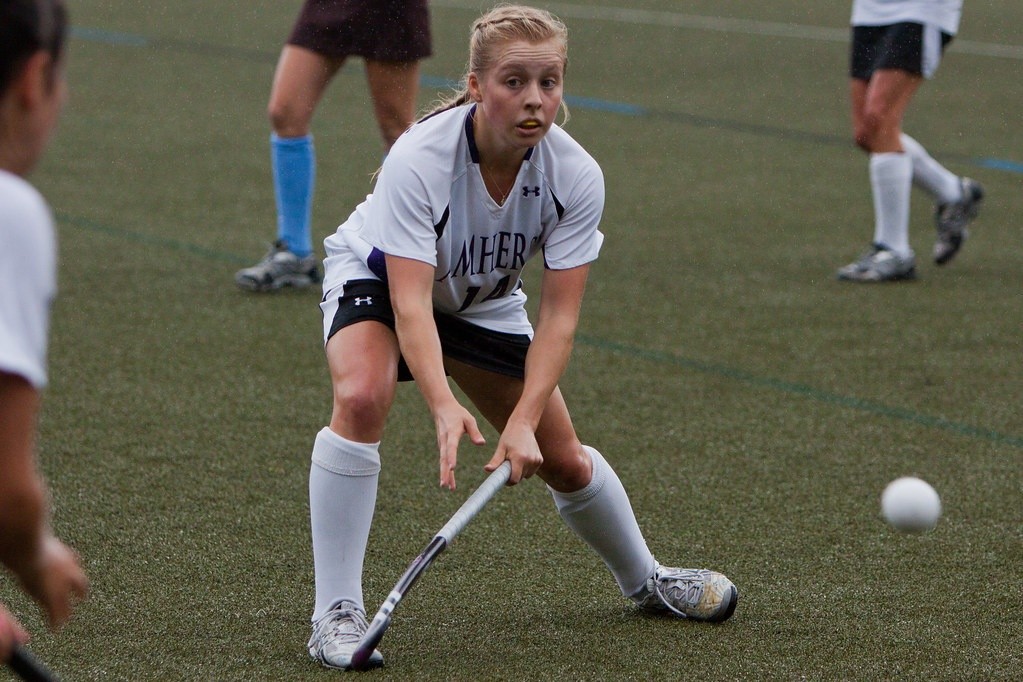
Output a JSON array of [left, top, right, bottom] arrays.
[[233, 1, 438, 297], [303, 6, 739, 669], [0, 0, 87, 667], [830, 1, 985, 284]]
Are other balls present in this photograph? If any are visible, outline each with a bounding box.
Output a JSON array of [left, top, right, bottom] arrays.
[[881, 477, 942, 531]]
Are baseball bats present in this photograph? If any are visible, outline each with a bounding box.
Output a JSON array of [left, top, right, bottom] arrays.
[[352, 458, 513, 665]]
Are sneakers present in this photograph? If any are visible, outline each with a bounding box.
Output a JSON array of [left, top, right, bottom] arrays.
[[307, 601, 385, 671], [836, 242, 918, 282], [232, 239, 321, 293], [932, 176, 995, 265], [629, 553, 738, 622]]
